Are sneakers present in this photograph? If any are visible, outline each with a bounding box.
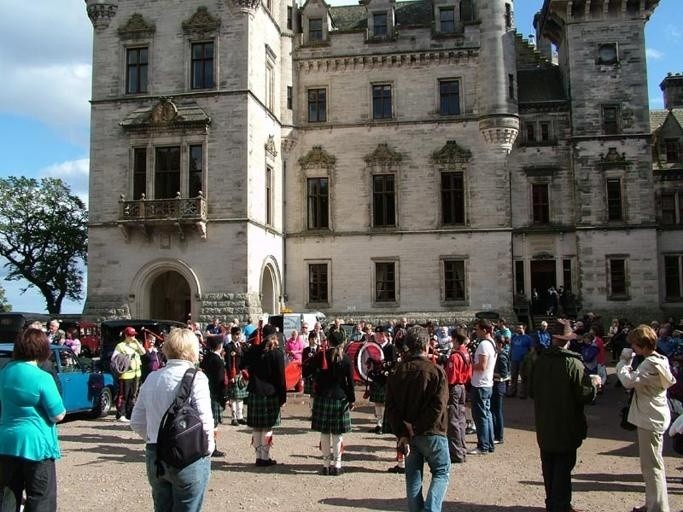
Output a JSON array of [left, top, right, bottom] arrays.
[[231, 418, 241, 426], [116, 415, 132, 423], [238, 418, 250, 425], [367, 420, 506, 475], [631, 502, 648, 512], [332, 465, 347, 476], [211, 449, 227, 457], [254, 458, 276, 467], [320, 467, 333, 476]]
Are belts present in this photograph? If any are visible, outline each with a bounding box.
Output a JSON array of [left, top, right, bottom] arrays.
[[144, 443, 162, 451]]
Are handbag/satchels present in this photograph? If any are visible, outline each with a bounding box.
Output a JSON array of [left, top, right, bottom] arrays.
[[618, 404, 640, 432]]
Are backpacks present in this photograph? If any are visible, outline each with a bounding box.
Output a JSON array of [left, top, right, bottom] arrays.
[[155, 366, 210, 479]]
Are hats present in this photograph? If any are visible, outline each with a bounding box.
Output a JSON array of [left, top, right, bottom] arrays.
[[543, 316, 584, 342], [649, 319, 660, 325], [124, 317, 394, 350]]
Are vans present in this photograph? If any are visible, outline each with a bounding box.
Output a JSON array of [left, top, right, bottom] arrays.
[[268, 310, 328, 339]]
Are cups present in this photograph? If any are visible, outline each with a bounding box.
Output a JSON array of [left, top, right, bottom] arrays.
[[621, 347, 633, 362], [590, 374, 600, 386]]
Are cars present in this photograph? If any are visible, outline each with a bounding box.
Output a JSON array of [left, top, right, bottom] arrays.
[[0, 311, 196, 427]]
